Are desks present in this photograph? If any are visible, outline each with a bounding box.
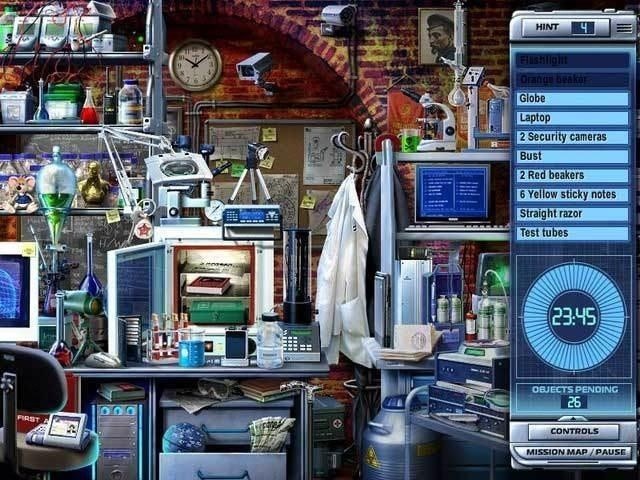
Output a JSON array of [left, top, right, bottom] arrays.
[[57, 347, 336, 479]]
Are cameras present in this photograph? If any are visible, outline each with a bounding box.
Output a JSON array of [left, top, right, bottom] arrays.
[[246, 140, 271, 164]]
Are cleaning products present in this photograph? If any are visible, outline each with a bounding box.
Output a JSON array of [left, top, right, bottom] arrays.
[[437, 294, 449, 323], [449, 294, 461, 323]]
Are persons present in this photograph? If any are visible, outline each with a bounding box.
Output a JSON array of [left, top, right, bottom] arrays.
[[426, 14, 456, 64], [64, 425, 75, 433]]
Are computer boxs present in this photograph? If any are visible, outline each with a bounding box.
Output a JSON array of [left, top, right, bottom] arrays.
[[95, 403, 138, 479]]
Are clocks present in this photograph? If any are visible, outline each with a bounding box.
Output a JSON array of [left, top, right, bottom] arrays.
[[168, 38, 225, 93]]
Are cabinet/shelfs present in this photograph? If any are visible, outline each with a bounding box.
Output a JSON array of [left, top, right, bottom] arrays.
[[1, 2, 166, 218], [105, 220, 280, 369], [372, 137, 511, 401]]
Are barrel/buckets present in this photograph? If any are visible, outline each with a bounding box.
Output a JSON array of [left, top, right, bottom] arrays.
[[362, 394, 439, 480]]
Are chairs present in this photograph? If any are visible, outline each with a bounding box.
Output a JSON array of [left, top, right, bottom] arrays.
[[1, 344, 101, 479]]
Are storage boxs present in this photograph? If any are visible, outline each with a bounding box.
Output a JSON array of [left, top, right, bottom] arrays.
[[311, 395, 346, 443]]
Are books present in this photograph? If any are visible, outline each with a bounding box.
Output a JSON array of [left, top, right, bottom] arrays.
[[187, 278, 231, 295], [377, 324, 442, 363], [241, 380, 296, 402]]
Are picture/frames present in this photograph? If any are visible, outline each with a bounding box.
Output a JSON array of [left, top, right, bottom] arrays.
[[416, 7, 469, 68]]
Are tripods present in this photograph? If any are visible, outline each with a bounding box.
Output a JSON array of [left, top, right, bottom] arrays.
[[229, 167, 274, 203]]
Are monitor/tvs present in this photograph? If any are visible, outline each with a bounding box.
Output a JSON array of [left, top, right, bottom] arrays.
[[414, 162, 491, 219], [0, 242, 41, 343]]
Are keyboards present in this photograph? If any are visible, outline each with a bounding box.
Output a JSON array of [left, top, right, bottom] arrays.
[[406, 223, 510, 232]]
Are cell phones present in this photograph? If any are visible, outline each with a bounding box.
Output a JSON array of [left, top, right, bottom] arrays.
[[225, 329, 247, 359]]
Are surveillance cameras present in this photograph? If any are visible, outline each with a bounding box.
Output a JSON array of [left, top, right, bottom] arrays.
[[321, 5, 358, 29], [235, 51, 275, 83]]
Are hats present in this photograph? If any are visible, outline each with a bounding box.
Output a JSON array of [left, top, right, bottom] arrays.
[[426, 14, 455, 38]]
